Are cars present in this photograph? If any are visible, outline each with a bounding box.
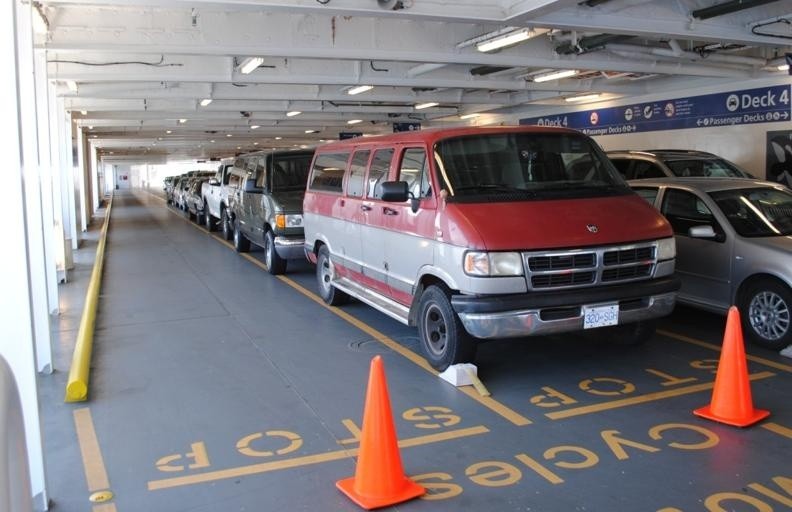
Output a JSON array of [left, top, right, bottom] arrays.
[[163, 147, 316, 277], [564, 149, 757, 184], [624, 178, 792, 353]]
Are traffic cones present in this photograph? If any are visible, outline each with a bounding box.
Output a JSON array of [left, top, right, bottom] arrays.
[[693, 305, 771, 427], [335, 354, 428, 511]]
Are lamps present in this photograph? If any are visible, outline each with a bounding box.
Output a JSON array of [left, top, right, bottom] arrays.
[[534, 69, 581, 82], [200, 98, 213, 107], [196, 134, 232, 149], [274, 136, 282, 140], [565, 94, 601, 104], [65, 81, 101, 150], [287, 110, 301, 117], [148, 118, 188, 149], [293, 133, 383, 149], [236, 142, 260, 149], [250, 124, 261, 130], [415, 102, 439, 110], [477, 27, 553, 53], [304, 129, 315, 134], [461, 113, 483, 119], [240, 57, 263, 74], [348, 118, 363, 125], [348, 85, 374, 94]]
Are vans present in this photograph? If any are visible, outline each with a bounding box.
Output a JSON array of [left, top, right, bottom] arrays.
[[300, 126, 682, 373]]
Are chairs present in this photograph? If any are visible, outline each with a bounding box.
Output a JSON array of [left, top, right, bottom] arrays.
[[528, 143, 569, 184], [710, 198, 759, 236]]
